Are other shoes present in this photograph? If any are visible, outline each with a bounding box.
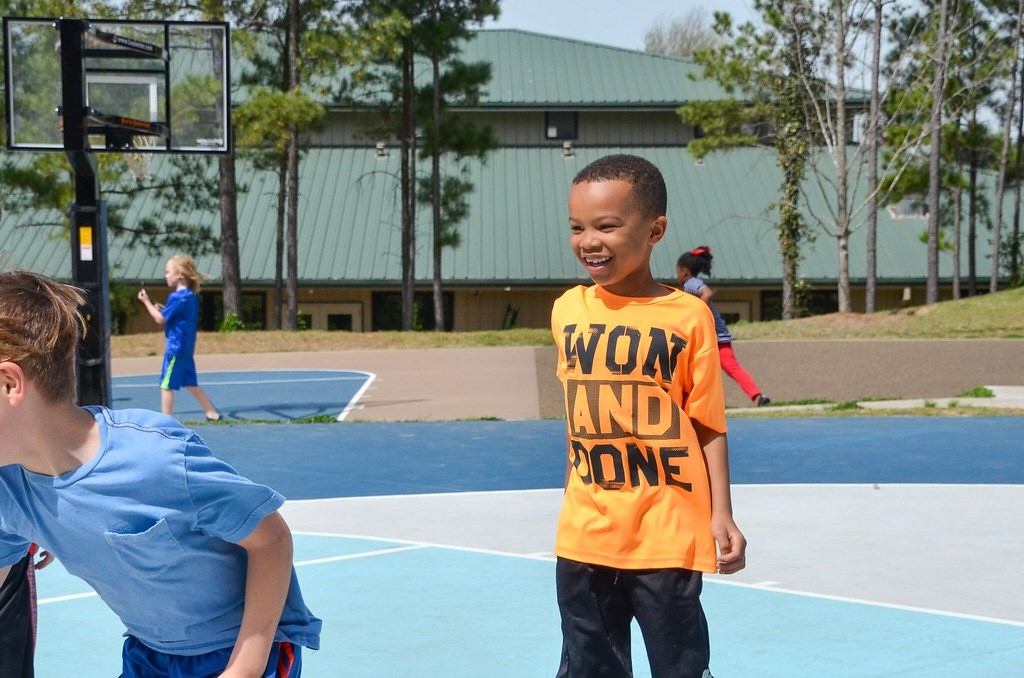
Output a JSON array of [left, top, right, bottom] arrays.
[[758, 395, 771, 409]]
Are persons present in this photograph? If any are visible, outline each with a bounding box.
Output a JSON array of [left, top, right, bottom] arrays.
[[552, 153, 747, 678], [1, 270, 321, 678], [138, 250, 220, 423], [676, 245, 770, 407]]
